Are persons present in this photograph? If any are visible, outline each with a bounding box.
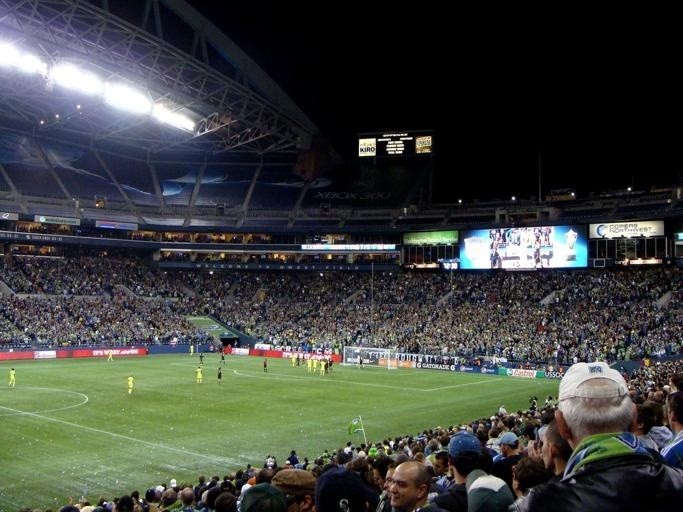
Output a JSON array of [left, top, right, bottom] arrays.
[[2, 220, 683, 512]]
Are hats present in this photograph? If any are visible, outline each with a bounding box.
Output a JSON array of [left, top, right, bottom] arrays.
[[493, 431, 520, 446], [558, 361, 630, 402], [315, 467, 379, 512], [271, 468, 318, 501], [240, 483, 290, 512], [466, 470, 514, 511], [448, 428, 482, 459], [171, 478, 176, 487], [156, 485, 165, 492]]
